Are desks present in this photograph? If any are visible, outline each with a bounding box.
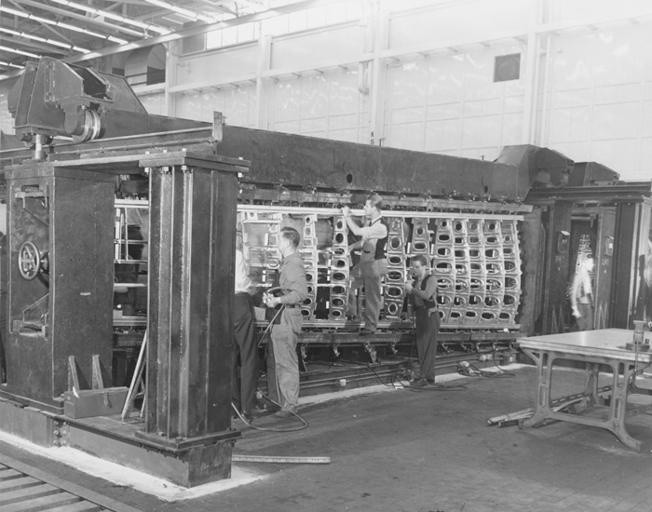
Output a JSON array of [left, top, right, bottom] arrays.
[[516, 327, 651, 449]]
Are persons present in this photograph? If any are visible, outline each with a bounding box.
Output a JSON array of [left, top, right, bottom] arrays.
[[399, 255, 439, 390], [571, 257, 593, 331], [260, 226, 309, 419], [335, 190, 390, 338], [231, 245, 260, 420]]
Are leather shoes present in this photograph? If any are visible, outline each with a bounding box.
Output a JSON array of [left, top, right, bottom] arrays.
[[275, 408, 298, 418], [407, 377, 437, 387]]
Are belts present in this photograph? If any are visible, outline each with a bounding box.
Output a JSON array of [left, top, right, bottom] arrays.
[[285, 303, 300, 309]]
